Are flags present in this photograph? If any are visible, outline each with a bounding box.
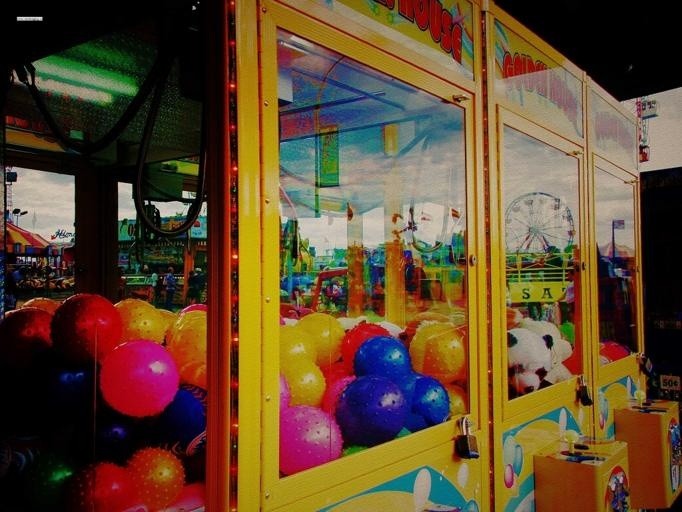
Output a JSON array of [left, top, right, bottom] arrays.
[[613, 218, 626, 230], [451, 208, 460, 219]]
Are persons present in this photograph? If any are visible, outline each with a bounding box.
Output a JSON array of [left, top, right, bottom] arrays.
[[183, 270, 196, 309], [150, 270, 164, 306], [192, 267, 206, 304], [163, 266, 177, 307]]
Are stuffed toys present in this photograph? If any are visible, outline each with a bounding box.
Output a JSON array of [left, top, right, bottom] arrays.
[[507, 308, 572, 402]]
